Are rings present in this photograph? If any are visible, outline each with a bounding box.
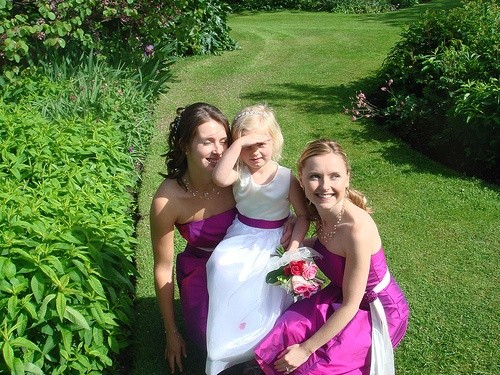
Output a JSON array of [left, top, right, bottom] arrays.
[[285, 366, 290, 372]]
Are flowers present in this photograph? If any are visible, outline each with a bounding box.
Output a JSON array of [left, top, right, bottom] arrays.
[[266, 245, 324, 302]]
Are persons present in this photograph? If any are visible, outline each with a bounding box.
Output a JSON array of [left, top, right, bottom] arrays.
[[205, 101, 310, 375], [149, 102, 238, 373], [255, 138, 408, 375]]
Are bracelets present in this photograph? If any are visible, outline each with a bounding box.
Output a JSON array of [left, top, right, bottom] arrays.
[[164, 328, 181, 335]]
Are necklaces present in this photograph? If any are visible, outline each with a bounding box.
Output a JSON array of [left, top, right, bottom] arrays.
[[182, 175, 228, 200], [315, 205, 346, 245]]
